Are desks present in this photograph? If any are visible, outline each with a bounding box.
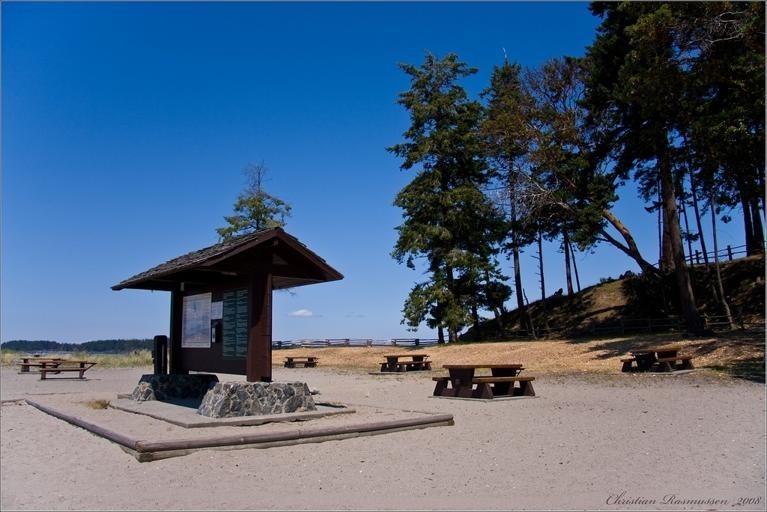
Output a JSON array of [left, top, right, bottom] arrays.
[[631, 349, 681, 372], [285, 357, 316, 368], [39, 360, 87, 379], [384, 354, 427, 372], [443, 364, 524, 398], [20, 358, 61, 371]]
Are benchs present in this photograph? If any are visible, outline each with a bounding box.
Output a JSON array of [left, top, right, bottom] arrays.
[[472, 377, 535, 399], [39, 368, 87, 379], [282, 361, 318, 368], [396, 361, 432, 372], [620, 358, 640, 372], [659, 356, 695, 372], [431, 376, 493, 397], [16, 362, 59, 374], [379, 363, 400, 372]]
